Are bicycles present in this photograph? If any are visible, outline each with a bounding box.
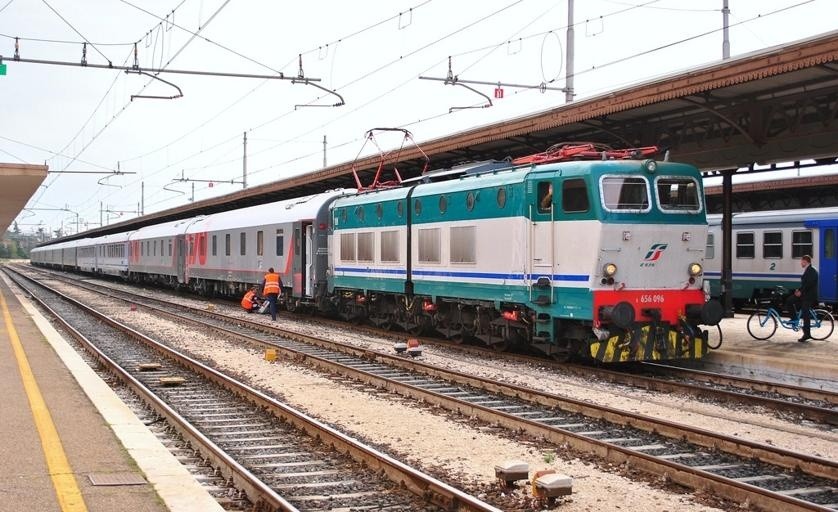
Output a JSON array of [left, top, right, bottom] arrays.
[[747, 286, 835, 342]]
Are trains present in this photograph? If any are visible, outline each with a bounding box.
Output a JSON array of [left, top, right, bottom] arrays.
[[27, 143, 726, 365], [698, 206, 838, 312]]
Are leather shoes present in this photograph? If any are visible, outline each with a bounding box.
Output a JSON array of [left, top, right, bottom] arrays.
[[787, 316, 799, 326], [799, 334, 811, 344]]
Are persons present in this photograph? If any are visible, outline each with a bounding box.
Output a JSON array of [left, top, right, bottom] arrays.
[[787, 255, 817, 342], [262, 268, 284, 321], [241, 287, 264, 312]]
